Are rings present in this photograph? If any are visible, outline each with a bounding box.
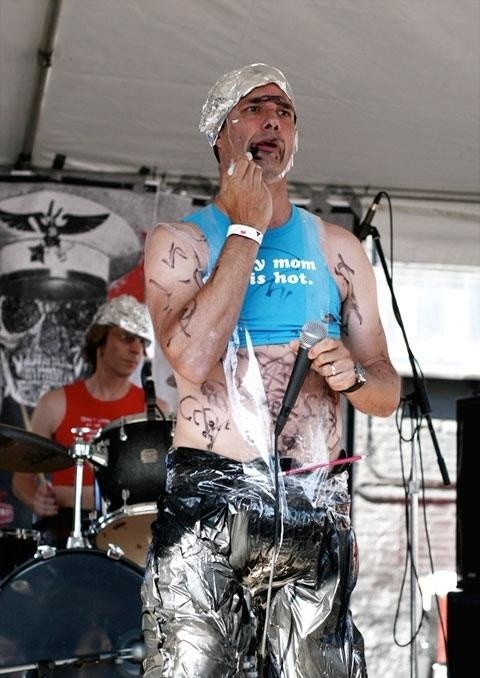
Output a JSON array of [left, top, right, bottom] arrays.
[[331, 365, 338, 376]]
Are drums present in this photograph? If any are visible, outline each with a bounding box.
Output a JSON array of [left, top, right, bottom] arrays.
[[0, 526, 42, 585], [0, 543, 145, 678], [89, 411, 177, 568]]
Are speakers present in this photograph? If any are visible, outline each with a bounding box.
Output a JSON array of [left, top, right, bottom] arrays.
[[454, 394, 480, 590], [446, 589, 480, 678]]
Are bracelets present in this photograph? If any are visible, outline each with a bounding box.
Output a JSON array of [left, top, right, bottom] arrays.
[[226, 224, 264, 245]]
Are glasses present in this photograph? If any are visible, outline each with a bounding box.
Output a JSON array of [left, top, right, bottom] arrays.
[[110, 330, 151, 346]]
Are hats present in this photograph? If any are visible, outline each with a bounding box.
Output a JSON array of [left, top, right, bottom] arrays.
[[92, 293, 154, 349], [199, 62, 296, 148], [1, 189, 142, 300]]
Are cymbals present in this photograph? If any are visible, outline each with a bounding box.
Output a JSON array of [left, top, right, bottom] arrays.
[[0, 423, 75, 472]]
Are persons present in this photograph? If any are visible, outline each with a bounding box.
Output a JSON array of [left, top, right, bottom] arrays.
[[10, 293, 171, 547], [141, 61, 402, 678]]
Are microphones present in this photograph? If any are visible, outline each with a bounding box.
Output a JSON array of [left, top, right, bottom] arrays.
[[274, 323, 327, 439], [357, 195, 382, 242]]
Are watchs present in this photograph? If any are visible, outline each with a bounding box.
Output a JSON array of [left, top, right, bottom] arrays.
[[341, 359, 368, 394]]
